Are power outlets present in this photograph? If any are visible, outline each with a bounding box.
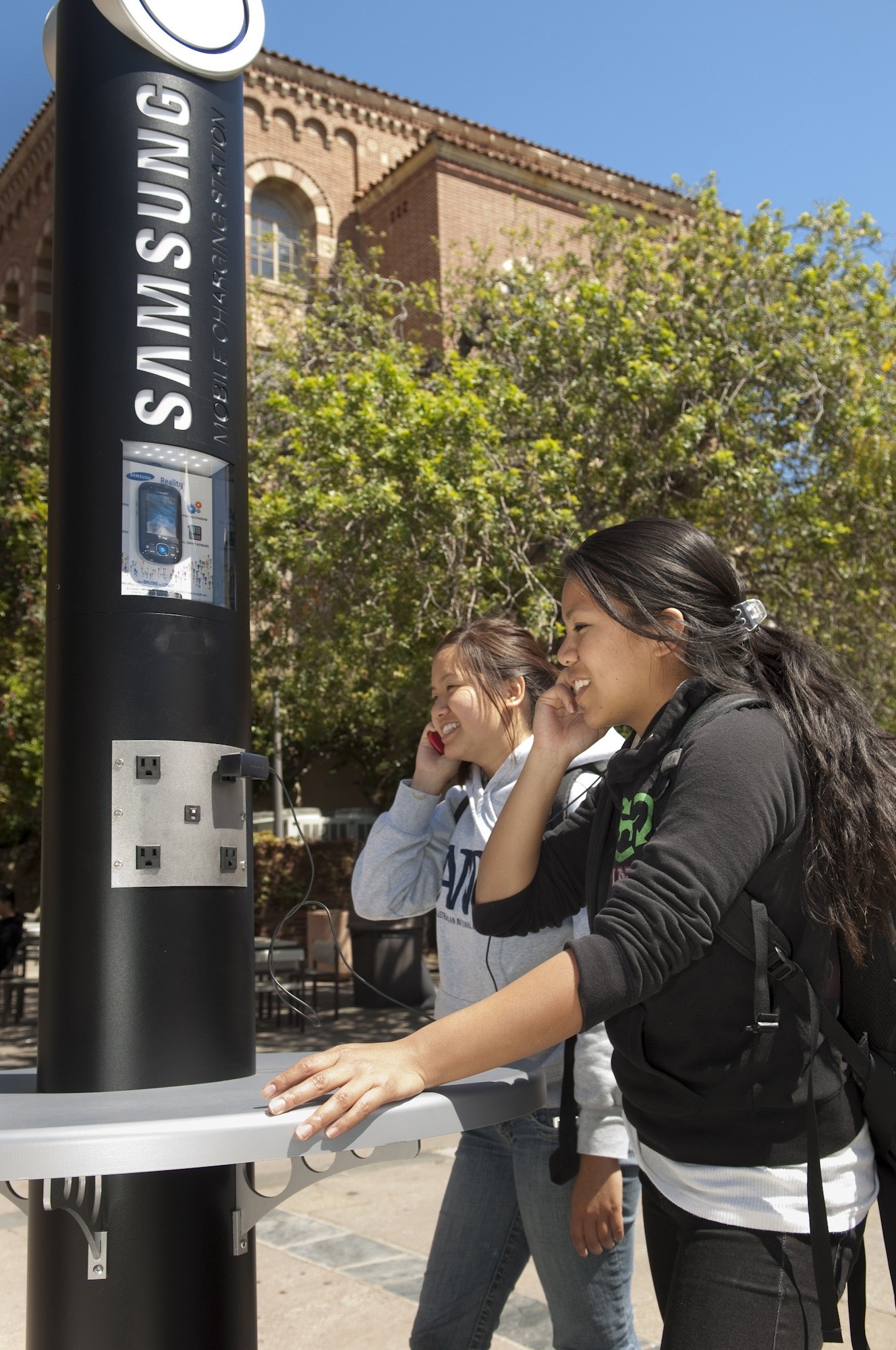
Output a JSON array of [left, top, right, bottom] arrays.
[[218, 760, 237, 784], [136, 755, 161, 780], [136, 845, 161, 870], [220, 846, 237, 871]]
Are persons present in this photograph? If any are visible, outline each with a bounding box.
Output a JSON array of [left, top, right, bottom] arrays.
[[350, 616, 646, 1350], [264, 519, 895, 1350], [0, 888, 26, 972]]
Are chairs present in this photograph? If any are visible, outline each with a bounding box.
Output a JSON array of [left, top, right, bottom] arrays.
[[0, 940, 40, 1024], [288, 940, 341, 1022], [255, 949, 306, 1030]]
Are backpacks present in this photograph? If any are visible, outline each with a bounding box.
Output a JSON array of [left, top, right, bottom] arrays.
[[585, 688, 896, 1350]]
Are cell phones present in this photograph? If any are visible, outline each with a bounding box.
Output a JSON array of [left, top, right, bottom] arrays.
[[138, 481, 183, 564], [427, 726, 445, 755]]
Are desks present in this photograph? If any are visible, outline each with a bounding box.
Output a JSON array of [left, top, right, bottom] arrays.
[[254, 937, 300, 1020], [14, 923, 41, 1025]]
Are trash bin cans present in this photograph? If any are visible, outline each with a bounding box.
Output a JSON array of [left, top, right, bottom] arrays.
[[347, 910, 424, 1010]]
